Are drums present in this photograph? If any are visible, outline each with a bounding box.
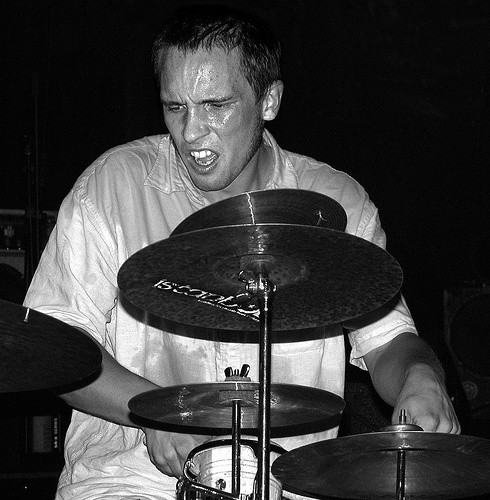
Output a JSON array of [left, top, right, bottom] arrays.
[[177, 435, 291, 499]]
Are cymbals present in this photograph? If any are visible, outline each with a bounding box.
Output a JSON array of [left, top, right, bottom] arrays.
[[116, 219, 406, 344], [271, 423, 490, 500], [1, 297, 104, 395], [127, 381, 347, 432], [166, 186, 349, 238]]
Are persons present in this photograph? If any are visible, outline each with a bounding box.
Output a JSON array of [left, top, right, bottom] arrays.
[[23, 5, 461, 500]]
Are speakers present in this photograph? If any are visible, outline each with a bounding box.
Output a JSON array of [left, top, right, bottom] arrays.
[[445, 282, 490, 411]]
